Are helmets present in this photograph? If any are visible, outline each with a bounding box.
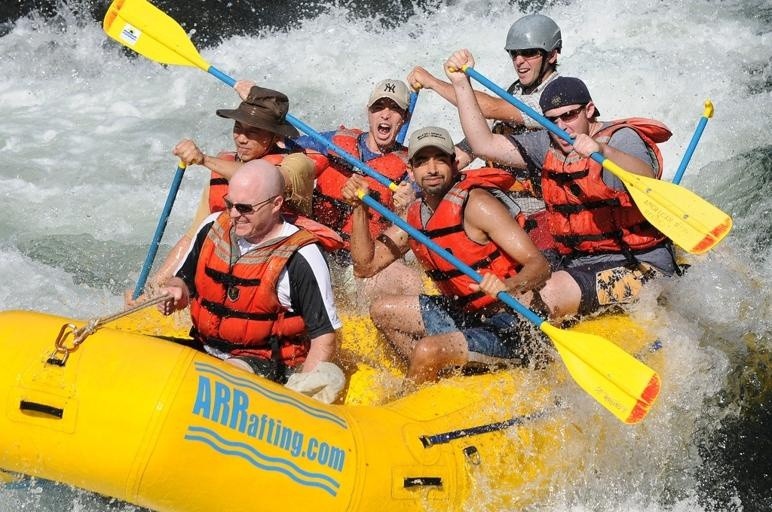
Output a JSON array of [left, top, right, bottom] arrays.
[[504, 12, 561, 54]]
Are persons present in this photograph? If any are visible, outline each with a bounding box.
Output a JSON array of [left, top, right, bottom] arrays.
[[153, 161, 345, 404], [235, 78, 409, 269], [443, 49, 676, 328], [341, 126, 551, 405], [407, 14, 563, 216], [123, 85, 315, 325]]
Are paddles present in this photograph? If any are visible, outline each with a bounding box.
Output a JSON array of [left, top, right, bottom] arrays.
[[359, 179, 661, 426], [104, 0, 398, 192], [448, 58, 732, 255]]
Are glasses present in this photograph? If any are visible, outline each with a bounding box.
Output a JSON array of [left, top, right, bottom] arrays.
[[508, 50, 540, 59], [547, 105, 584, 122], [222, 191, 279, 213]]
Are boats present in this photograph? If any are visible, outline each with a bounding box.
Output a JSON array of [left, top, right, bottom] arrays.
[[1, 253, 771, 512]]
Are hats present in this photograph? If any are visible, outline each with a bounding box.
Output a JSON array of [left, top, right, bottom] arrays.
[[408, 127, 455, 162], [368, 79, 411, 112], [217, 86, 300, 138], [539, 76, 600, 117]]
